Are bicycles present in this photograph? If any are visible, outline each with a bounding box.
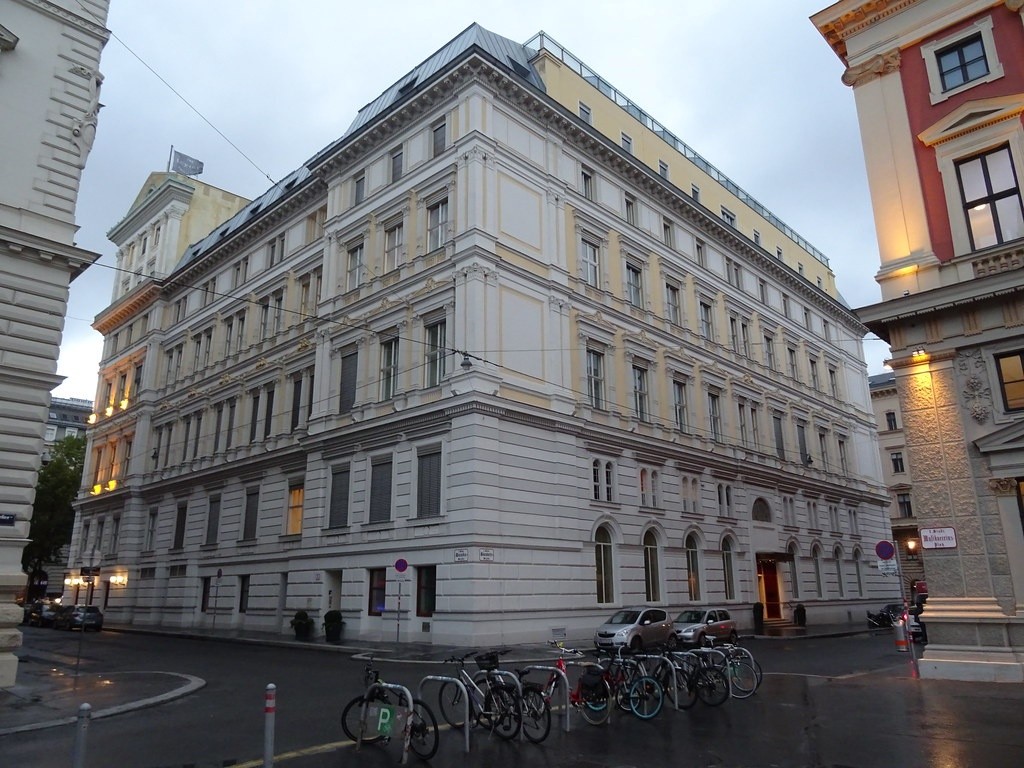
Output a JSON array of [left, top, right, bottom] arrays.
[[440, 646, 762, 760], [342, 653, 439, 760]]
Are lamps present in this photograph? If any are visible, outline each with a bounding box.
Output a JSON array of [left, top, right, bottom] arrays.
[[460, 354, 472, 370], [906, 538, 921, 555], [807, 455, 813, 465], [63, 579, 81, 588], [151, 450, 159, 460], [912, 346, 926, 357], [110, 576, 125, 586]]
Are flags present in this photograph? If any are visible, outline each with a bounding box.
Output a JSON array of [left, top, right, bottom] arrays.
[[172, 150, 204, 175]]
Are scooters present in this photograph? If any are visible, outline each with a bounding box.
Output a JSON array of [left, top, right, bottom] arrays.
[[866, 609, 897, 629]]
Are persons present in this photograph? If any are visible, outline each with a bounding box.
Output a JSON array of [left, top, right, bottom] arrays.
[[15, 597, 45, 627]]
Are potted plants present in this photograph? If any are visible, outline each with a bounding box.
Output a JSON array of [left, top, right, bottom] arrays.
[[793, 603, 807, 626], [321, 611, 346, 643], [752, 601, 765, 634], [290, 610, 315, 642]]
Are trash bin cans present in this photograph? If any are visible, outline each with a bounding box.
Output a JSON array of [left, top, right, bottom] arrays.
[[289, 610, 314, 642], [321, 610, 347, 644], [891, 616, 910, 652]]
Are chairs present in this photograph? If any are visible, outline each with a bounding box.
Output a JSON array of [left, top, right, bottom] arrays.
[[694, 613, 701, 622], [708, 615, 714, 620]]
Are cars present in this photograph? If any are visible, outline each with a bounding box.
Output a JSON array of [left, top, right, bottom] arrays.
[[673, 607, 738, 648], [51, 604, 103, 633], [904, 606, 923, 637], [880, 604, 906, 623], [26, 603, 65, 629], [594, 607, 678, 654]]
